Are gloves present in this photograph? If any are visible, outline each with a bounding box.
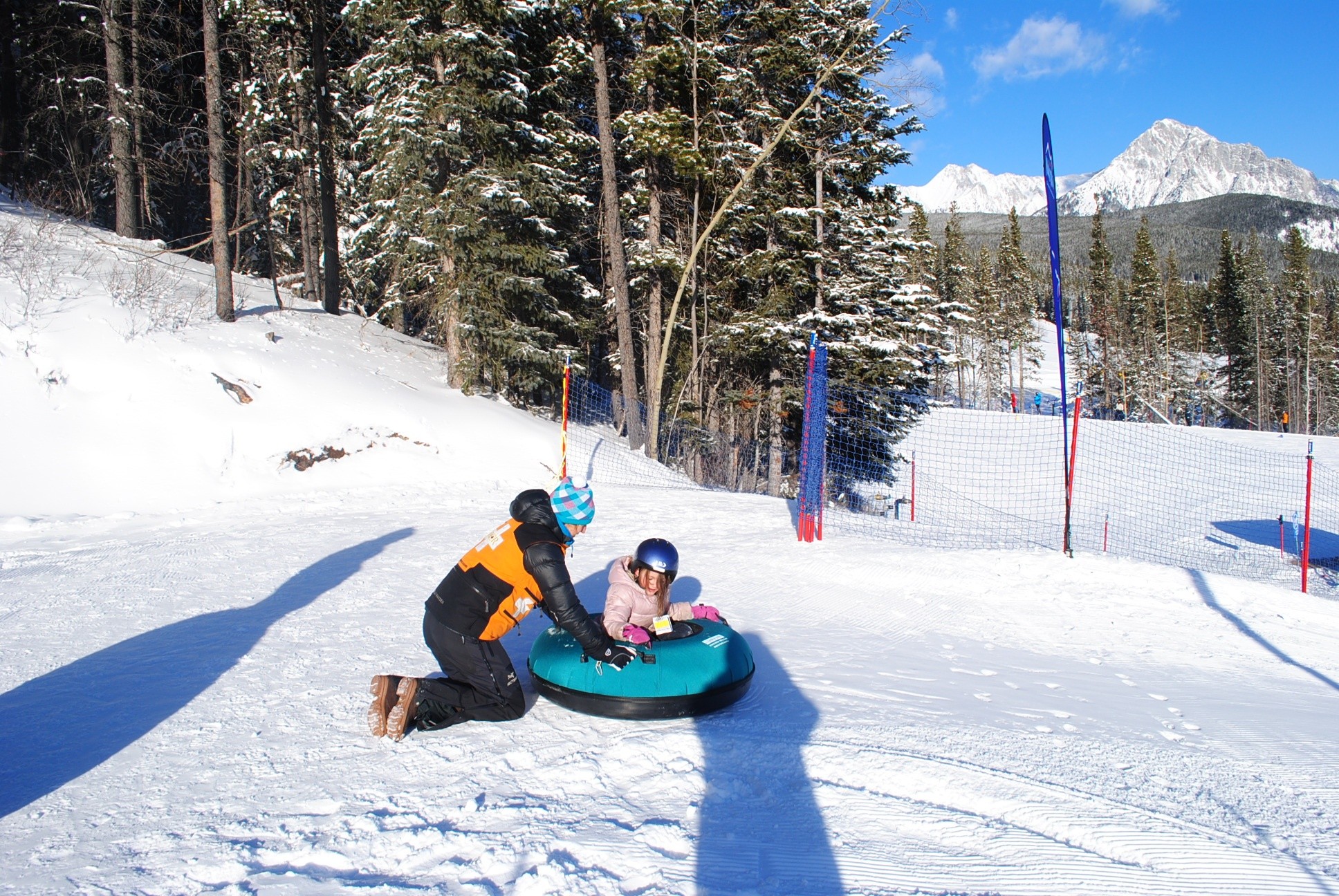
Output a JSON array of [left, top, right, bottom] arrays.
[[622, 623, 652, 650], [691, 603, 723, 624], [606, 644, 638, 672]]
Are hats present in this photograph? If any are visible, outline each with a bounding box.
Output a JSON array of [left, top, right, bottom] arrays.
[[549, 476, 595, 525]]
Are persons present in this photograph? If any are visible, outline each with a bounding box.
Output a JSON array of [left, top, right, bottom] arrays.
[[366, 472, 639, 744], [602, 537, 722, 650]]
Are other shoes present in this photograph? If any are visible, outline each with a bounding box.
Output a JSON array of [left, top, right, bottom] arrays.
[[387, 677, 426, 742], [367, 674, 405, 737]]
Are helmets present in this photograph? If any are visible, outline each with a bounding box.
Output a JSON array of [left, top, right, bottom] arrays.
[[631, 538, 679, 584]]
